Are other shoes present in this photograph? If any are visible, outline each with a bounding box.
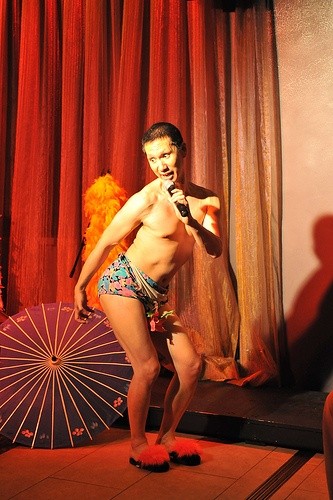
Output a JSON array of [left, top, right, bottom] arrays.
[[158, 438, 202, 466], [128, 445, 170, 472]]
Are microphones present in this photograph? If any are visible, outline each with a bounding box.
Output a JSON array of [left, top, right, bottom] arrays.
[[165, 180, 188, 217]]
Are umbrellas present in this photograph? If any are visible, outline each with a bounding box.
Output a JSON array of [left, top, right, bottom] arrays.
[[0, 301, 134, 451]]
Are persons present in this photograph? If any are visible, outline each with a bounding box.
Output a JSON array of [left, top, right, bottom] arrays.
[[73, 122, 223, 474]]
